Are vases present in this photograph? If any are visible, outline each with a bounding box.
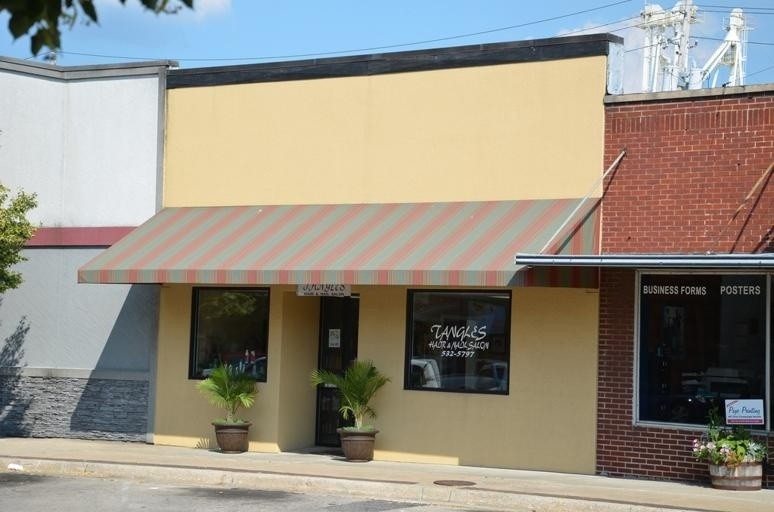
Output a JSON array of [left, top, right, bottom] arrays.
[[710, 457, 763, 491]]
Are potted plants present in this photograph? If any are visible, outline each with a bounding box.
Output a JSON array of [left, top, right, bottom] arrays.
[[193, 350, 258, 454], [307, 358, 393, 461]]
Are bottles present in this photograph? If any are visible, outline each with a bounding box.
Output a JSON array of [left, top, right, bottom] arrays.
[[243, 349, 256, 364], [320, 352, 339, 435]]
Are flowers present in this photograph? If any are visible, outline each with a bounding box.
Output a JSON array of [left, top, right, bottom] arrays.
[[691, 414, 769, 467]]
